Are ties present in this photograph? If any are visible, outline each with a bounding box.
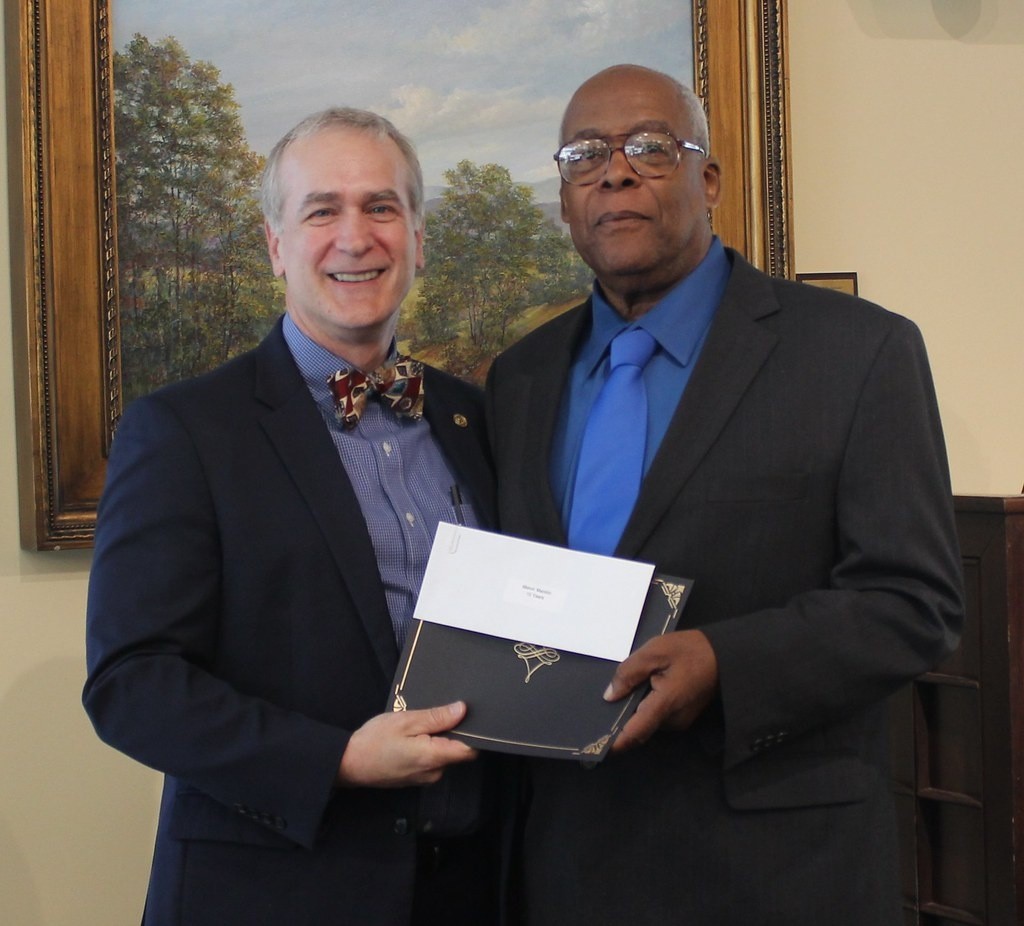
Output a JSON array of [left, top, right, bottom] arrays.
[[568, 331, 657, 557]]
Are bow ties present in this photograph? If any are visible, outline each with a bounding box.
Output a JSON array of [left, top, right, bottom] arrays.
[[327, 358, 426, 431]]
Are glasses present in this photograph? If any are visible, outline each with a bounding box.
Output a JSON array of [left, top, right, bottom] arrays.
[[554, 128, 707, 187]]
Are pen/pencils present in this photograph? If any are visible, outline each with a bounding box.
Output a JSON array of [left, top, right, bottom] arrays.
[[449, 486, 470, 526]]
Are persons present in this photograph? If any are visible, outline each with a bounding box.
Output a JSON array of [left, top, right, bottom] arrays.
[[83, 107, 501, 926], [487, 64, 965, 926]]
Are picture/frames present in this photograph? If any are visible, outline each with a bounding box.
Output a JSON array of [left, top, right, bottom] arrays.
[[5, 0, 796, 552]]
